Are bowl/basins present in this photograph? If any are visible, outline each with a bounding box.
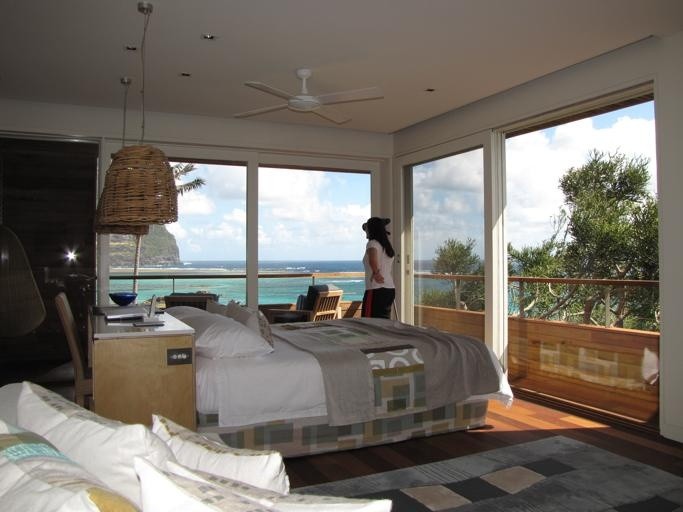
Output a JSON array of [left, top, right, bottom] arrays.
[[108, 292, 137, 306]]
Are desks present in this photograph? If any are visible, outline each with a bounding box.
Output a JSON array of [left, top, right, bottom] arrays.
[[82, 307, 195, 431]]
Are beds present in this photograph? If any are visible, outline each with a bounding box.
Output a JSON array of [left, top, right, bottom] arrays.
[[196, 316, 514, 461]]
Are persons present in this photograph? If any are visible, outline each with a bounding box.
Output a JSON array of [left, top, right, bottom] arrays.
[[361, 217, 396, 319]]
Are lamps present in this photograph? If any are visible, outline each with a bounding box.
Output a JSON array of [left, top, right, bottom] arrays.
[[95, 2, 178, 228], [95, 77, 150, 236]]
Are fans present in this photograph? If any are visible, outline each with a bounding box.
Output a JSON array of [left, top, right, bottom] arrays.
[[233, 66, 384, 125]]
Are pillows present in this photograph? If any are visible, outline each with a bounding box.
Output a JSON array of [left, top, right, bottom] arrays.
[[151, 413, 291, 494], [162, 297, 276, 360], [165, 458, 392, 511], [132, 457, 273, 512], [18, 383, 175, 506]]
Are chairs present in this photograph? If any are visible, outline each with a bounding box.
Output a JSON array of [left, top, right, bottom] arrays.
[[164, 292, 219, 311], [270, 283, 343, 324], [54, 292, 93, 407]]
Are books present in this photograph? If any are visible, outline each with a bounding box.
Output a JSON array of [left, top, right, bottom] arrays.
[[103, 306, 147, 320], [133, 320, 164, 327]]
